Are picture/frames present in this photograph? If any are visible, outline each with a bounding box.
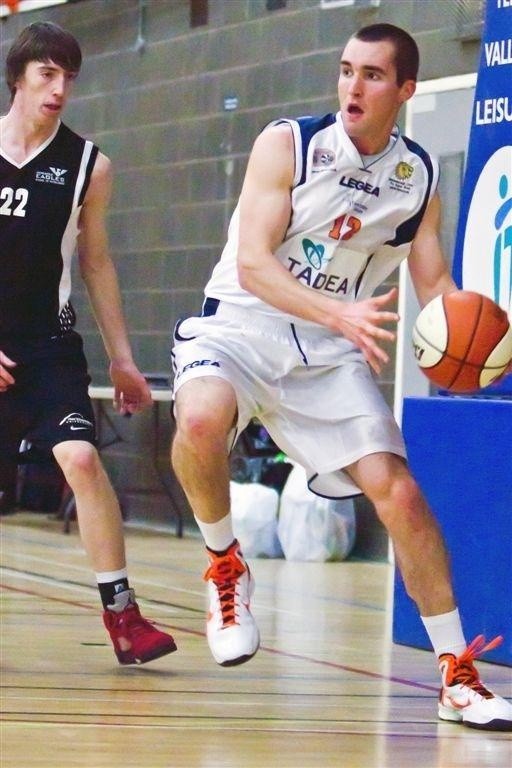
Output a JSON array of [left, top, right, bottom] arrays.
[[57, 386, 175, 534]]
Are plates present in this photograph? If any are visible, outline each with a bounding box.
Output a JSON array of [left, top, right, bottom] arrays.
[[393, 2, 512, 668]]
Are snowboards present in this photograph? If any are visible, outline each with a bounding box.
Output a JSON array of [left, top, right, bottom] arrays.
[[412, 291, 512, 393]]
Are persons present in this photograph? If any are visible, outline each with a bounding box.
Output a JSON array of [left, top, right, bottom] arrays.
[[2, 20, 178, 666], [169, 22, 512, 734]]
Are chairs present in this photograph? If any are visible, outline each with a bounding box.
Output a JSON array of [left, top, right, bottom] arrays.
[[437, 651, 512, 730], [203, 538, 261, 667], [103, 587, 177, 665]]
